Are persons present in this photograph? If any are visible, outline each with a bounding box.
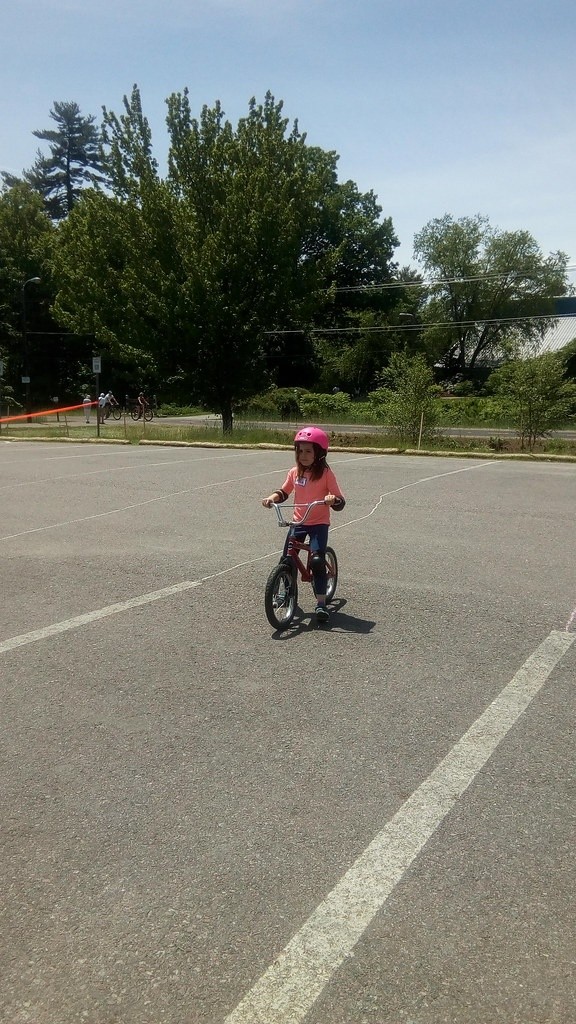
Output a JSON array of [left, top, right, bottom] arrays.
[[99, 393, 105, 424], [83, 395, 91, 424], [262, 427, 346, 621], [105, 391, 119, 419], [136, 392, 149, 420]]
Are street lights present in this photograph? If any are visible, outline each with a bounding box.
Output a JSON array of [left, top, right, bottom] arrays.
[[22, 277, 41, 423]]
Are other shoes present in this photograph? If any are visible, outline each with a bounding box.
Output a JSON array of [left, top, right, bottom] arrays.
[[274, 598, 286, 607], [316, 595, 326, 608]]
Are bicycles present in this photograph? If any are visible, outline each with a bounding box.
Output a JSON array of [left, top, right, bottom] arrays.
[[101, 403, 122, 420], [131, 401, 154, 422], [264, 499, 340, 630]]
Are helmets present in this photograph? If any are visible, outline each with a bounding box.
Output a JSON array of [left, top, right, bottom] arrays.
[[293, 427, 329, 453]]
